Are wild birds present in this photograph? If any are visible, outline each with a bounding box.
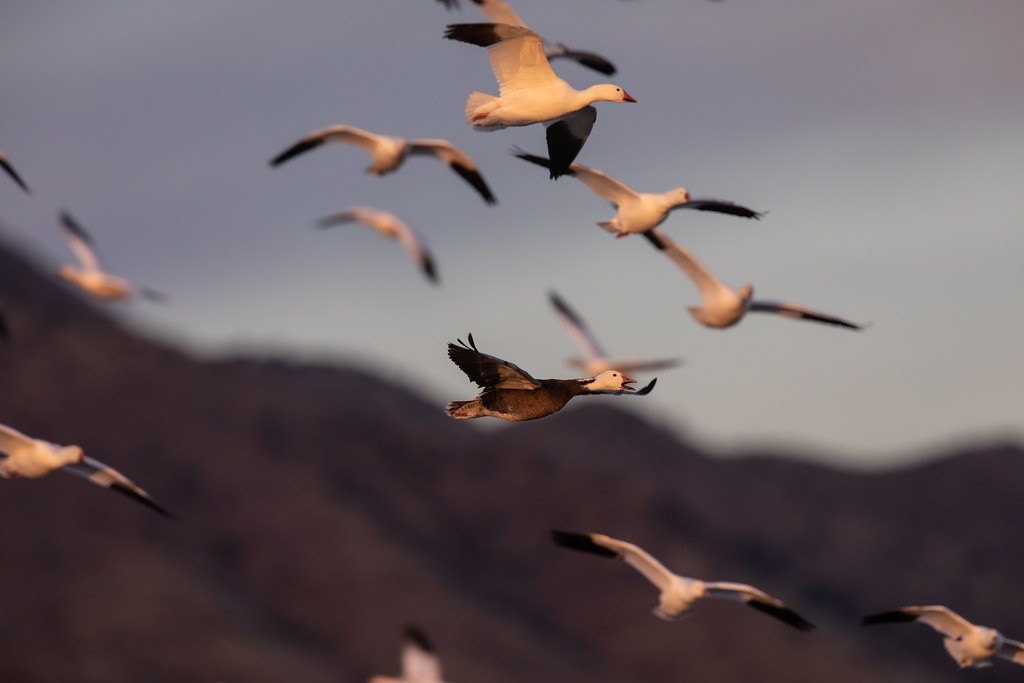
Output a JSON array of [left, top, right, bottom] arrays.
[[366, 622, 447, 683], [0, 152, 28, 190], [643, 228, 862, 331], [442, 22, 637, 180], [861, 605, 1024, 670], [435, 0, 617, 76], [547, 292, 683, 376], [0, 421, 173, 518], [508, 144, 766, 239], [550, 529, 818, 635], [316, 210, 437, 281], [445, 332, 658, 423], [55, 214, 168, 304], [267, 124, 497, 207]]
